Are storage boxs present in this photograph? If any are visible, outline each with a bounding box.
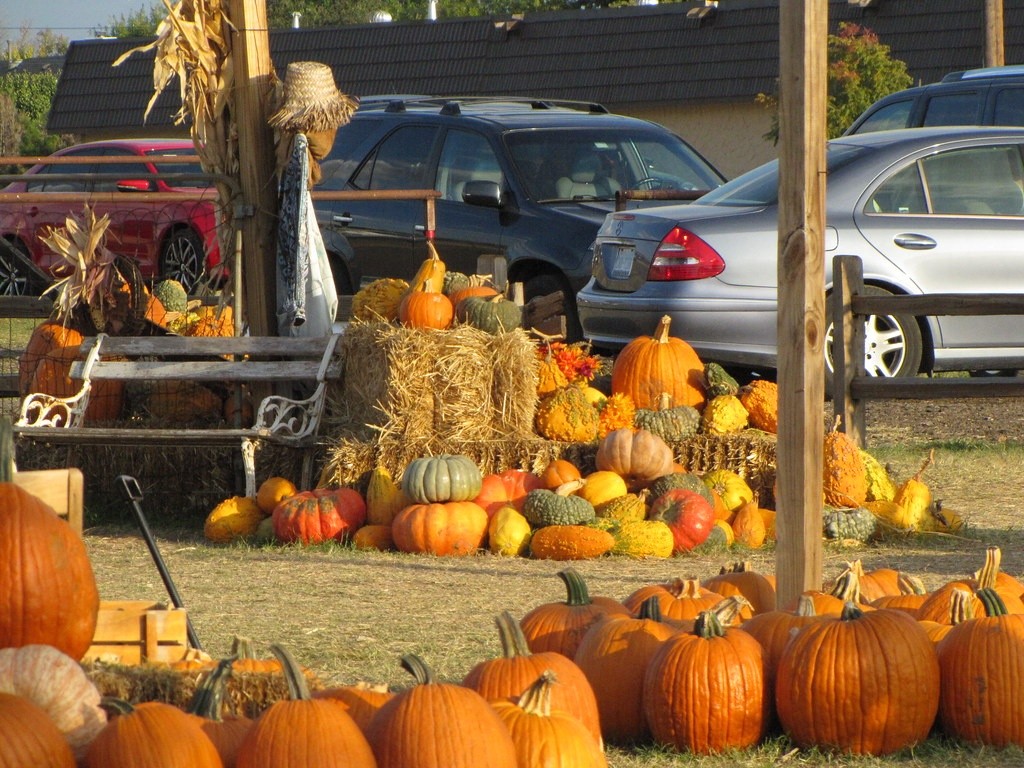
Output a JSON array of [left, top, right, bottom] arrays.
[[81, 598, 182, 662]]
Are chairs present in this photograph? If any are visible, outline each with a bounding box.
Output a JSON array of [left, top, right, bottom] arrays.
[[944, 163, 994, 215], [15, 468, 84, 538], [455, 159, 505, 204], [556, 152, 625, 203]]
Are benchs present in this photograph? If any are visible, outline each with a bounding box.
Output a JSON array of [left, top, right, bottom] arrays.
[[14, 330, 341, 499]]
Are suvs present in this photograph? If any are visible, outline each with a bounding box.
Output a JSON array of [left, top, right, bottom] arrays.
[[840, 65, 1024, 190], [312, 93, 731, 346]]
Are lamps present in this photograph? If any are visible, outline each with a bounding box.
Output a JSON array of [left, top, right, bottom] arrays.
[[848, 0, 871, 7], [495, 13, 523, 30], [687, 0, 720, 18]]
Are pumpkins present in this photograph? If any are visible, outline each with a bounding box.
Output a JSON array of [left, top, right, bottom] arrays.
[[520, 543, 1024, 755], [0, 482, 606, 768], [18, 242, 963, 561]]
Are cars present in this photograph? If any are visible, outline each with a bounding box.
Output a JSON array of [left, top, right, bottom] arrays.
[[575, 126, 1024, 398], [0, 138, 230, 297]]
